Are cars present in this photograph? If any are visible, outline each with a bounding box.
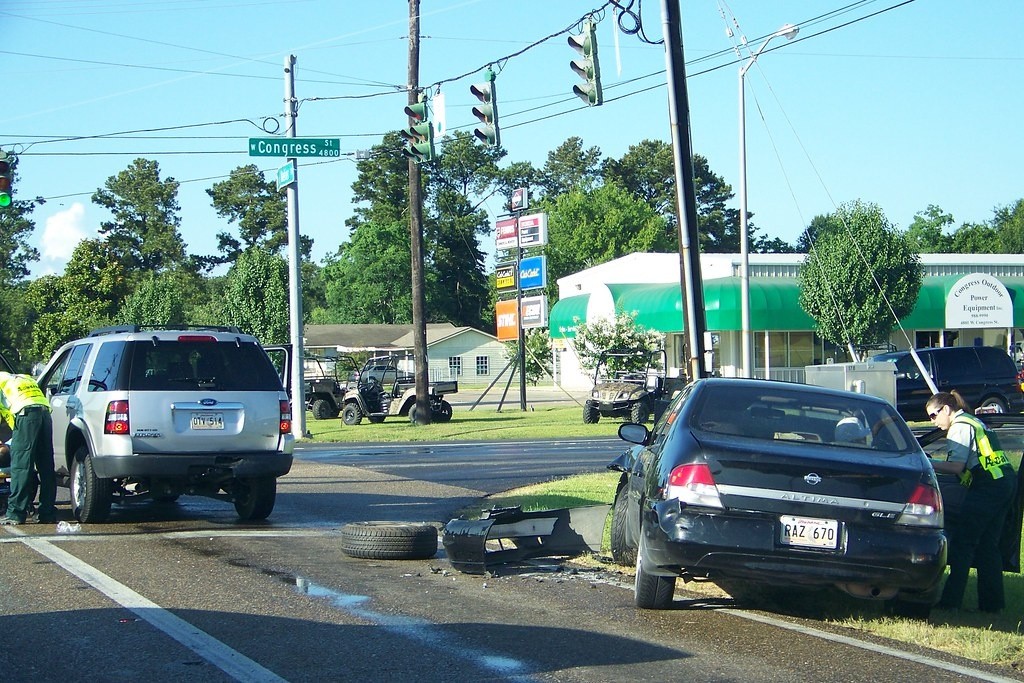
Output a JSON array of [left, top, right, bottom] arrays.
[[606, 377, 1023, 620], [348, 364, 414, 384]]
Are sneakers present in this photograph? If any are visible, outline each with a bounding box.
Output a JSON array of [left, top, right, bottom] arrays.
[[0, 517, 21, 525]]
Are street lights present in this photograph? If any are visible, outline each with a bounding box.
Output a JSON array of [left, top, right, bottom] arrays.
[[737, 20, 801, 380]]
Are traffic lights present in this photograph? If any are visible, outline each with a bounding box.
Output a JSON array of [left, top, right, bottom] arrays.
[[0, 161, 14, 209], [400, 129, 422, 165], [565, 24, 603, 106], [468, 80, 500, 147], [404, 102, 429, 124], [410, 123, 433, 163]]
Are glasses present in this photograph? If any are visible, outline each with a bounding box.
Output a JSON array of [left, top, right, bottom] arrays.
[[929, 406, 944, 418]]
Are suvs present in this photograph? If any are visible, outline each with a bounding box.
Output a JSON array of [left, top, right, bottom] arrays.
[[1, 321, 295, 525], [862, 345, 1024, 430]]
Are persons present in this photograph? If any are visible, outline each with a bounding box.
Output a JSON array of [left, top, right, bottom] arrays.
[[925, 389, 1018, 614], [835, 417, 871, 444], [0, 370, 57, 525]]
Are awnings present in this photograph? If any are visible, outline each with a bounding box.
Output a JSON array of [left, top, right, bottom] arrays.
[[549, 277, 1024, 338]]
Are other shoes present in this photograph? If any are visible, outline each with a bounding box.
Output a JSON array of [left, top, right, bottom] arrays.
[[930, 603, 959, 613], [965, 608, 1001, 617]]
